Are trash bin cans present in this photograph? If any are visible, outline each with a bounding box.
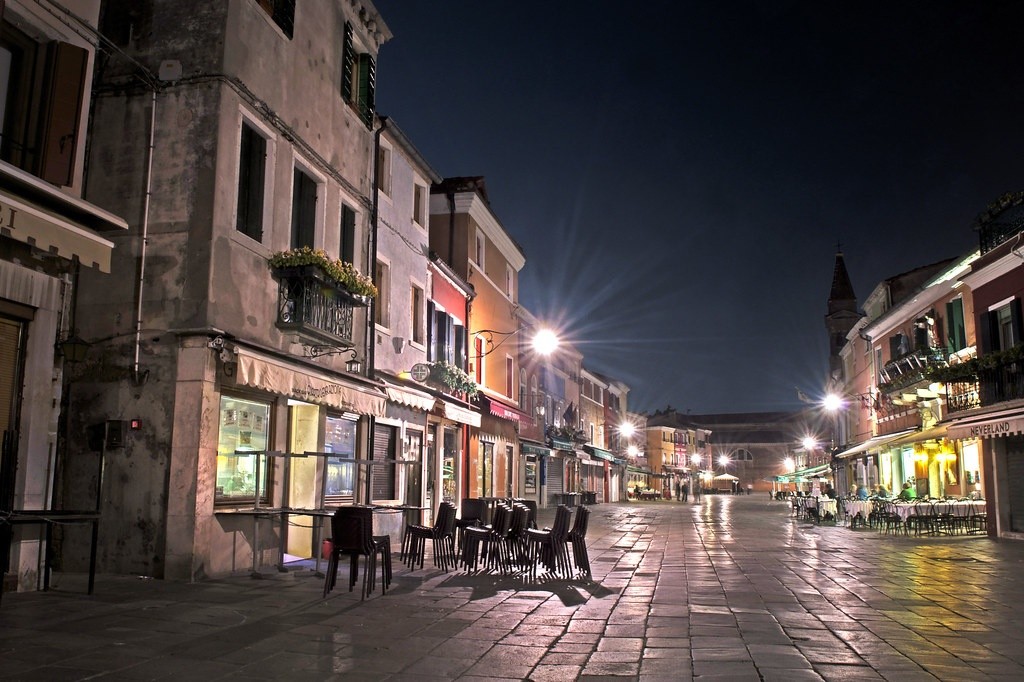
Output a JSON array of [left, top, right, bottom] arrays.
[[587, 490, 598, 504]]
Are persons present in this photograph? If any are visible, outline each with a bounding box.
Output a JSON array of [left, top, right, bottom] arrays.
[[673, 481, 701, 504], [823, 483, 921, 504]]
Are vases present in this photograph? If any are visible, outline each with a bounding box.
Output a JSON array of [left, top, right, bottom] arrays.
[[272, 267, 372, 307]]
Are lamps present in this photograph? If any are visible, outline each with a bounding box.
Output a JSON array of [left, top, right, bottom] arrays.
[[308, 343, 360, 373], [471, 321, 556, 360]]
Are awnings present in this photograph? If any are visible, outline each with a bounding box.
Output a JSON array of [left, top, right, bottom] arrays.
[[0, 190, 115, 275], [220, 340, 713, 480], [774, 398, 1024, 484]]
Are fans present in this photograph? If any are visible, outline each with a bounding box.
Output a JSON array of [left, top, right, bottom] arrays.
[[427, 360, 480, 401]]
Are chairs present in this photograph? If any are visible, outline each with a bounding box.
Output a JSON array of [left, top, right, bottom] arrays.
[[322, 504, 393, 601], [785, 491, 986, 535], [399, 497, 591, 576]]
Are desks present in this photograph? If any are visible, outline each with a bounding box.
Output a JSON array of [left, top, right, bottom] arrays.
[[894, 501, 986, 522], [842, 500, 875, 520], [790, 496, 837, 523]]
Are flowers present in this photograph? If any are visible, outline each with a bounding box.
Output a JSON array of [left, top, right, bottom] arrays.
[[561, 425, 589, 443], [267, 246, 379, 305], [546, 426, 561, 436]]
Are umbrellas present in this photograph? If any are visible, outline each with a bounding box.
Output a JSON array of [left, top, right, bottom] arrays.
[[713, 473, 739, 489]]
